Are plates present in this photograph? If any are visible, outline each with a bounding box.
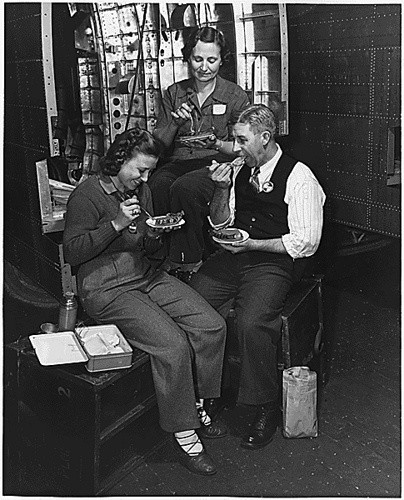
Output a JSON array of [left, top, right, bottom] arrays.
[[212, 227, 249, 244], [146, 215, 186, 229], [177, 132, 213, 141]]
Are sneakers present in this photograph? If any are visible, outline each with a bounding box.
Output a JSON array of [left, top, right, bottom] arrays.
[[205, 386, 236, 421]]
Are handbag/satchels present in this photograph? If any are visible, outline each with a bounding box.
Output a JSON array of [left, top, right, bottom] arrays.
[[282, 366, 318, 439]]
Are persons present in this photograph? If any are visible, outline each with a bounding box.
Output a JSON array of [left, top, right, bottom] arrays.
[[62, 128, 228, 476], [206, 104, 327, 450], [148, 24, 251, 285]]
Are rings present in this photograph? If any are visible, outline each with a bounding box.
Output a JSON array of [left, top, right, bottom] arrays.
[[132, 209, 137, 215]]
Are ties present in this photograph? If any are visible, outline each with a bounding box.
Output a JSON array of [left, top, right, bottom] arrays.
[[249, 167, 260, 192]]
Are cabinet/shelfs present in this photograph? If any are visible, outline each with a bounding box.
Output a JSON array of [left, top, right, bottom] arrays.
[[4, 318, 172, 495], [223, 273, 324, 407]]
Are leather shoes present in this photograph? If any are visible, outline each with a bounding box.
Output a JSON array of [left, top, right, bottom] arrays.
[[241, 400, 282, 448], [170, 428, 217, 475], [195, 399, 227, 438]]
